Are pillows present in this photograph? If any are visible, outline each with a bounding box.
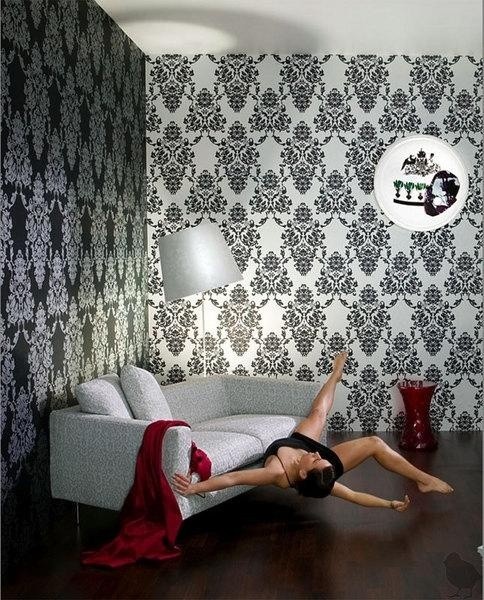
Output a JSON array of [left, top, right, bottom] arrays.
[[73, 363, 173, 422]]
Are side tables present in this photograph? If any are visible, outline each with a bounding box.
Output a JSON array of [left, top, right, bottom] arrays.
[[398, 379, 440, 451]]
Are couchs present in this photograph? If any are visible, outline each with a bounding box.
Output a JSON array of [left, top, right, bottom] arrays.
[[49, 376, 328, 527]]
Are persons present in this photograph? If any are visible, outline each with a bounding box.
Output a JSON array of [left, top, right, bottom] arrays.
[[168, 350, 454, 512], [425, 170, 462, 216]]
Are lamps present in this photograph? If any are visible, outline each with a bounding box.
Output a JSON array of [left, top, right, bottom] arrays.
[[158, 221, 245, 377]]
